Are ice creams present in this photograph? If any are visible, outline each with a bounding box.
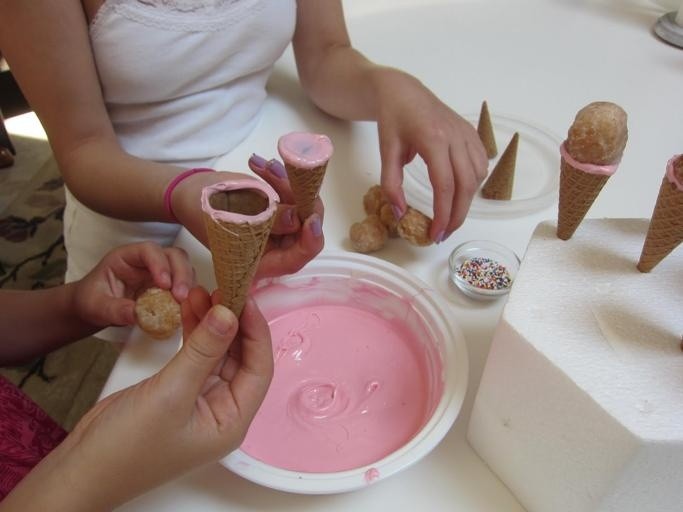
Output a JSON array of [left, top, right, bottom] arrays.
[[199, 178, 280, 319], [636, 155, 683, 274], [558, 102, 630, 242], [276, 129, 335, 224]]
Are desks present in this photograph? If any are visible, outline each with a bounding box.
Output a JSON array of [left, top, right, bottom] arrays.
[[99, 0, 682, 510]]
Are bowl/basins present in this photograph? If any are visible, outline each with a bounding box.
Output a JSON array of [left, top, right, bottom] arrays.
[[178, 248, 470, 497]]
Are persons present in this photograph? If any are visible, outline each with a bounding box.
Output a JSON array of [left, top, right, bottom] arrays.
[[0, 1, 491, 344], [0, 242, 274, 511]]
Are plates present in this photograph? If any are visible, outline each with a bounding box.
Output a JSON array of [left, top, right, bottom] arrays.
[[446, 239, 521, 302], [401, 107, 567, 222]]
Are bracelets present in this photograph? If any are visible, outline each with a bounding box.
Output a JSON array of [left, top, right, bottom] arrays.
[[163, 166, 215, 222]]
